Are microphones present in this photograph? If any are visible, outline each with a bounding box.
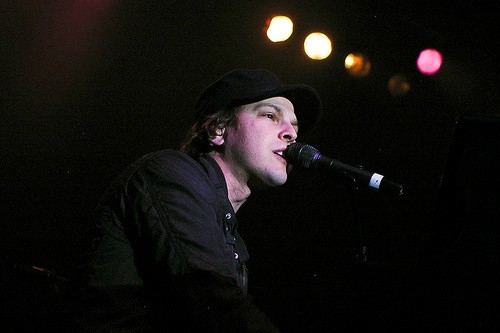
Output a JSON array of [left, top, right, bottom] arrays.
[[285, 142, 403, 197]]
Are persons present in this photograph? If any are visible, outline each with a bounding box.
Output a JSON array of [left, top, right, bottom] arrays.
[[42, 68, 299, 333]]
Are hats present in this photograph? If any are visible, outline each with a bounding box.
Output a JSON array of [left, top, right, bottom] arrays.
[[194, 67, 323, 141]]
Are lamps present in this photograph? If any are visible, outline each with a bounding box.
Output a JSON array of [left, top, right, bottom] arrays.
[[265, 12, 444, 105]]
